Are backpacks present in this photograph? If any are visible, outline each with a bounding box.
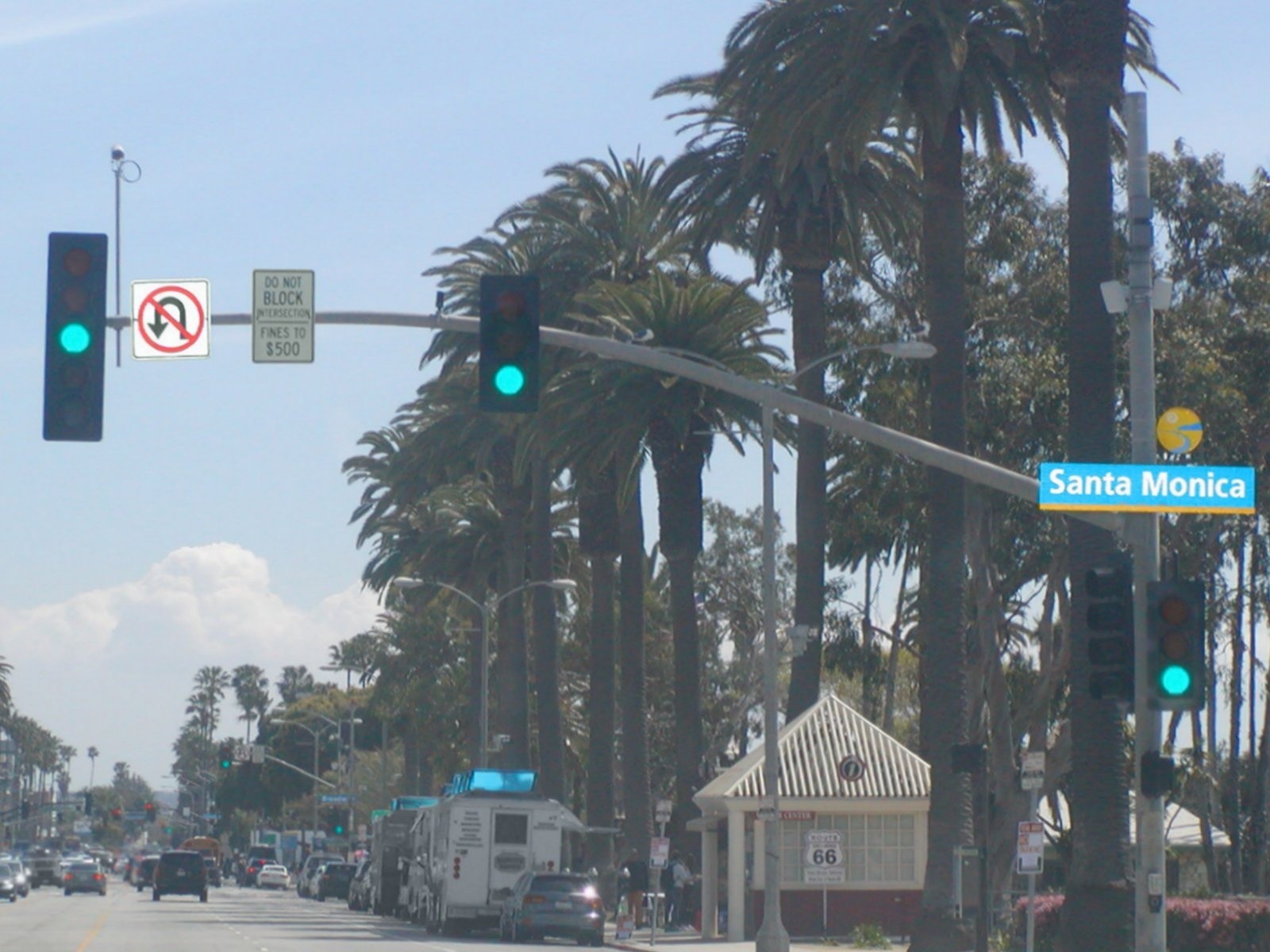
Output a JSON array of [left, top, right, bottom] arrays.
[[660, 864, 680, 890]]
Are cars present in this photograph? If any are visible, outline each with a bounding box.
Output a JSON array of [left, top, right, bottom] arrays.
[[308, 860, 358, 903], [179, 837, 291, 892], [0, 845, 159, 903], [64, 862, 109, 897]]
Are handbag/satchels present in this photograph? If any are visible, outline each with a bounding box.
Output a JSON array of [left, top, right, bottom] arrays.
[[640, 895, 653, 911]]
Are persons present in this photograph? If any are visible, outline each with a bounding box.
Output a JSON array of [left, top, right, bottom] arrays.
[[612, 848, 700, 931], [223, 849, 246, 879]]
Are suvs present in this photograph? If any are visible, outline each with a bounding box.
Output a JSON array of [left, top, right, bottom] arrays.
[[498, 869, 605, 948], [152, 849, 208, 903], [296, 854, 346, 899]]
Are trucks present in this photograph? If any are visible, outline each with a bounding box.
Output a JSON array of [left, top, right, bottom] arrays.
[[347, 767, 562, 936]]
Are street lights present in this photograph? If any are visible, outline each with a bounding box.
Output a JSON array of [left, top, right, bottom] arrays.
[[266, 705, 363, 850], [391, 576, 578, 771], [318, 663, 390, 810], [596, 341, 940, 952]]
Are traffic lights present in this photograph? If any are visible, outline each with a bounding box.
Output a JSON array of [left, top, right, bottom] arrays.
[[43, 231, 108, 443], [1146, 579, 1205, 709], [221, 743, 232, 768], [146, 803, 154, 822], [334, 809, 345, 835], [113, 806, 122, 821], [1085, 554, 1134, 702], [479, 274, 537, 414]]
